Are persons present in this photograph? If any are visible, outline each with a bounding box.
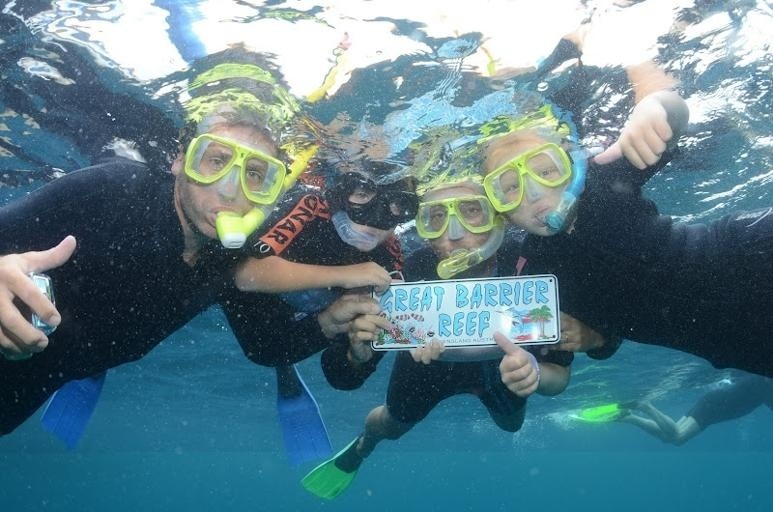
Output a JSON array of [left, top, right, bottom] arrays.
[[1, 55, 772, 497]]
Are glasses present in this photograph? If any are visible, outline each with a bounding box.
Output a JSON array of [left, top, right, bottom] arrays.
[[182, 133, 287, 207], [414, 193, 495, 241], [336, 171, 420, 232], [482, 143, 573, 213]]
